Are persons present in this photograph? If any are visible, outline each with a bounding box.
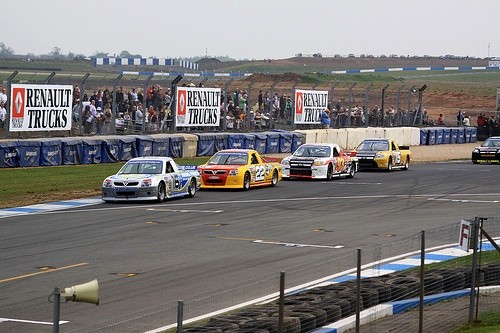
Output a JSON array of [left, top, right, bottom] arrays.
[[72, 80, 436, 136], [0, 87, 8, 108], [437, 114, 445, 124], [156, 163, 162, 173], [378, 144, 384, 150], [318, 149, 326, 155], [0, 101, 6, 128], [456, 110, 500, 127], [245, 155, 254, 163]]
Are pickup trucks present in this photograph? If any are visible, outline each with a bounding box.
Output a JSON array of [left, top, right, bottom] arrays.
[[281, 144, 358, 180], [102, 156, 202, 203], [353, 139, 411, 172], [196, 149, 282, 189], [472, 137, 500, 164]]
